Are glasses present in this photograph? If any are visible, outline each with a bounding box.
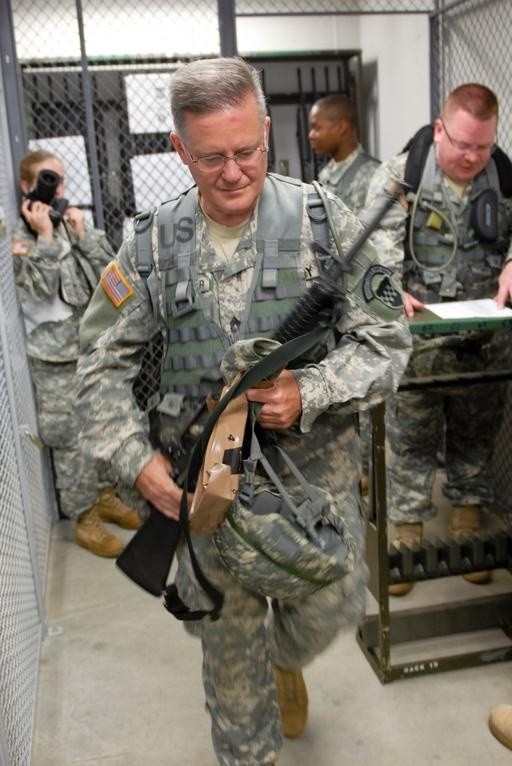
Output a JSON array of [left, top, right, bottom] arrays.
[[441, 117, 499, 157], [181, 125, 268, 174]]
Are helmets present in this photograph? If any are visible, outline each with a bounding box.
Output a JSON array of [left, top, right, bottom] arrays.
[[209, 470, 357, 603]]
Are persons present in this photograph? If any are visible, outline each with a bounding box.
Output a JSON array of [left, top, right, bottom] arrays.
[[11, 146, 146, 560], [364, 80, 512, 598], [69, 53, 416, 766], [301, 91, 390, 218], [482, 701, 510, 752]]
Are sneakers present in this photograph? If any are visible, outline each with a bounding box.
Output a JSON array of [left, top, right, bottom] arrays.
[[487, 703, 511, 752]]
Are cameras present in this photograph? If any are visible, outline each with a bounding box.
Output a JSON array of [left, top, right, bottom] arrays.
[[20, 169, 69, 227]]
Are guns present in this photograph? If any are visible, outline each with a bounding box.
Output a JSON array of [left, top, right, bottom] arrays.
[[114, 179, 412, 597]]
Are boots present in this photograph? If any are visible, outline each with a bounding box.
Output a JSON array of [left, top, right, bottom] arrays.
[[273, 662, 310, 739], [387, 522, 423, 597], [97, 487, 142, 530], [74, 502, 125, 559], [445, 504, 494, 586]]
